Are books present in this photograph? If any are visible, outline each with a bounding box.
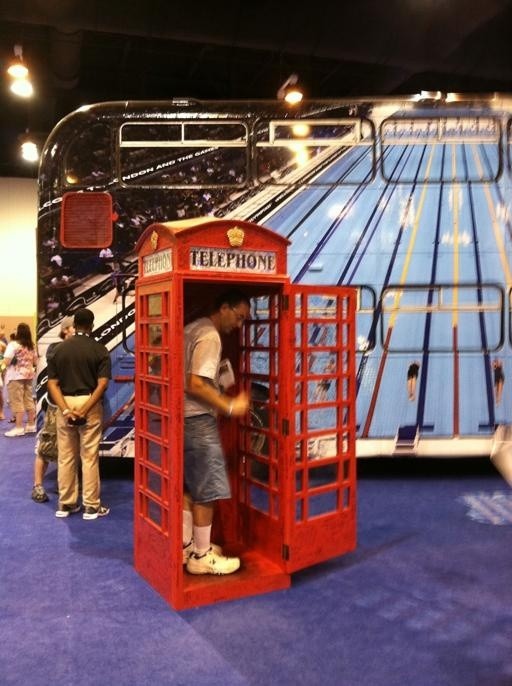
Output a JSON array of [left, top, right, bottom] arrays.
[[218, 358, 235, 390]]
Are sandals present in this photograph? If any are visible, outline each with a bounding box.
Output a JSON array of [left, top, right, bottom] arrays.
[[7, 413, 17, 424]]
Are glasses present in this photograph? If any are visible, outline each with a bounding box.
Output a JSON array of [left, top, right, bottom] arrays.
[[231, 305, 247, 324]]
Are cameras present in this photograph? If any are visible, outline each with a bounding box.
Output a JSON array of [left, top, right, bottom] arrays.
[[67, 416, 87, 426]]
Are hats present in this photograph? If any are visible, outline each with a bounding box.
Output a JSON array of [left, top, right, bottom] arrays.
[[60, 314, 76, 330]]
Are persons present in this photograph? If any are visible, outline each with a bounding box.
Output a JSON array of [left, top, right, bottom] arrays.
[[30, 317, 89, 504], [49, 308, 112, 521], [39, 133, 266, 310], [0, 322, 40, 438], [406, 361, 420, 401], [173, 296, 256, 574], [493, 358, 504, 407], [310, 358, 337, 403]]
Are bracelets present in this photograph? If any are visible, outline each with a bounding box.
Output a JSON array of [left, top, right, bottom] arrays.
[[62, 408, 71, 415], [228, 401, 233, 415]]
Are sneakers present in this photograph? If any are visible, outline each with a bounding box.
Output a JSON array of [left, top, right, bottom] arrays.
[[55, 503, 81, 518], [4, 427, 25, 436], [187, 548, 241, 576], [24, 424, 37, 433], [31, 485, 49, 503], [82, 505, 111, 520], [182, 541, 222, 565]]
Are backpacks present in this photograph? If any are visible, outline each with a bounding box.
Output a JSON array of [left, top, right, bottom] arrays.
[[39, 404, 59, 463]]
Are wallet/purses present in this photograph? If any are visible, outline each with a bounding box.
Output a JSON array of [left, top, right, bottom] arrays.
[[10, 356, 17, 365]]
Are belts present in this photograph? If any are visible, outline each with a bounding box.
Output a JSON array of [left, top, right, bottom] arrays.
[[63, 391, 92, 397]]
